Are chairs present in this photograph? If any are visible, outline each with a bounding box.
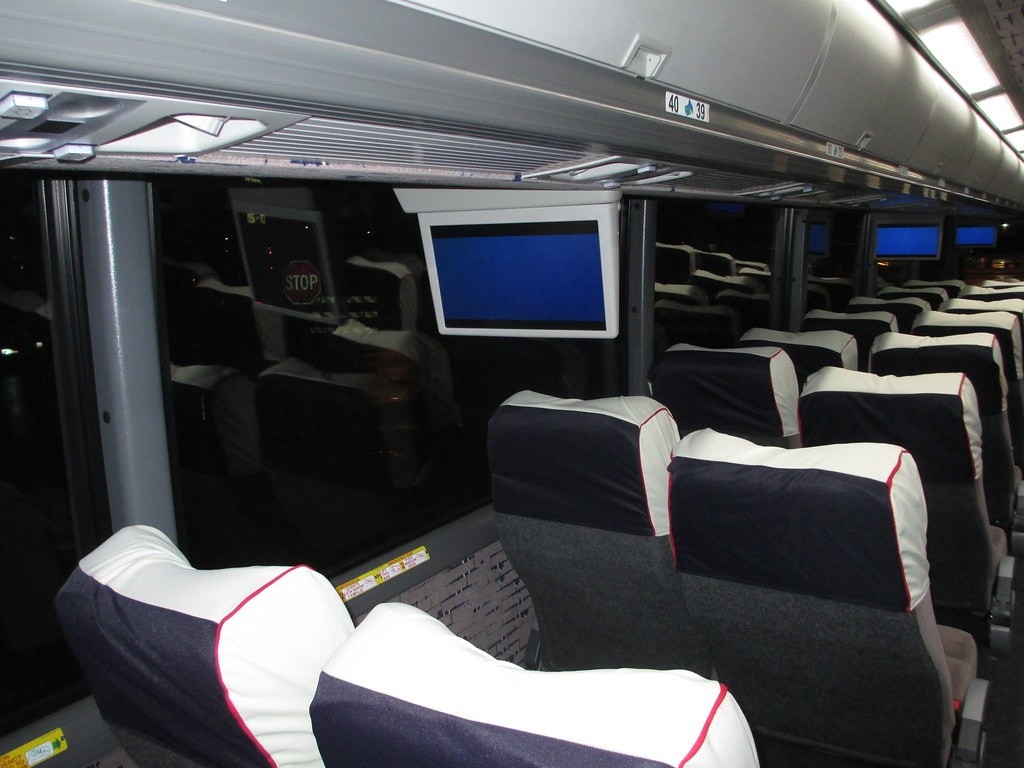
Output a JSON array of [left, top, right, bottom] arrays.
[[166, 256, 454, 526], [54, 522, 356, 768], [652, 241, 886, 343], [485, 278, 1024, 768], [308, 602, 760, 768]]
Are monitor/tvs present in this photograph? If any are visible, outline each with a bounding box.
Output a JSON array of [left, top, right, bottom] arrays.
[[873, 216, 944, 260], [232, 187, 339, 324], [954, 227, 996, 247], [393, 189, 620, 339], [808, 219, 831, 257]]
